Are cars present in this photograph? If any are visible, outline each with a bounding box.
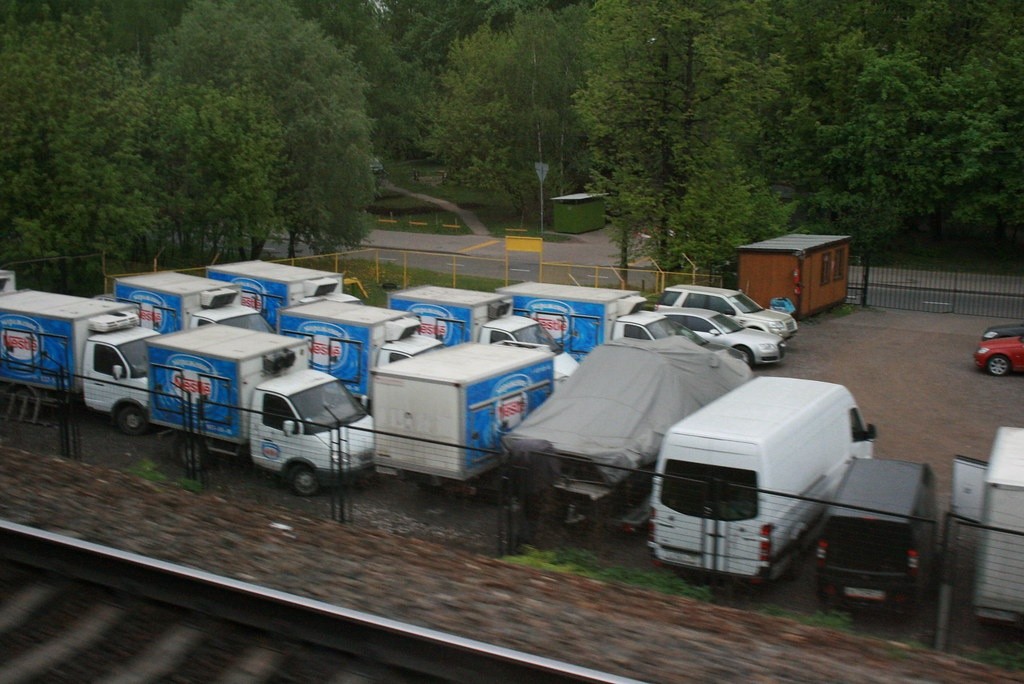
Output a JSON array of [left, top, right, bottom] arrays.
[[501, 335, 751, 516], [971, 322, 1024, 377]]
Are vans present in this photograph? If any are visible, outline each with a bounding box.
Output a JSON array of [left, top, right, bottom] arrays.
[[817, 456, 948, 618], [651, 373, 879, 584]]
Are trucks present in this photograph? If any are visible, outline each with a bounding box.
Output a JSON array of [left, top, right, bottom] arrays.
[[495, 278, 751, 364], [113, 259, 369, 337], [275, 282, 581, 504], [143, 323, 377, 491], [0, 288, 160, 436]]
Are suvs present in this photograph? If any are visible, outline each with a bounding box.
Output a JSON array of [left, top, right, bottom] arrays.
[[653, 282, 799, 366]]
